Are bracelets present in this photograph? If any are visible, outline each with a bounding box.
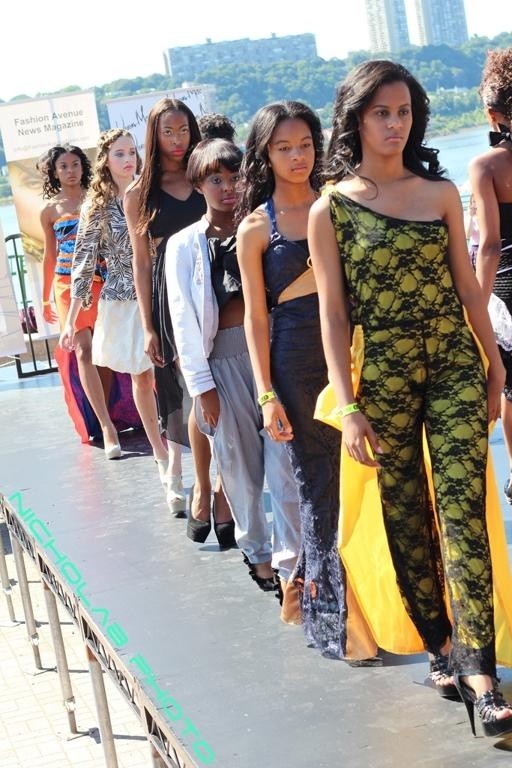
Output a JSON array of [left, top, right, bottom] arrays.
[[337, 402, 359, 418], [42, 300, 51, 306], [257, 392, 275, 407]]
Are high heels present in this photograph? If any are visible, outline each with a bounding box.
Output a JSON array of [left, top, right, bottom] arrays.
[[429, 650, 512, 738], [211, 503, 234, 549], [156, 452, 186, 516], [187, 485, 212, 543]]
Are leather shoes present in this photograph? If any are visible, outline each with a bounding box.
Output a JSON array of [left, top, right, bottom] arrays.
[[255, 571, 280, 593], [104, 444, 122, 459]]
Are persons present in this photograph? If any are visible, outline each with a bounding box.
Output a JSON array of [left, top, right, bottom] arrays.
[[196, 110, 236, 142], [39, 142, 125, 460], [165, 138, 306, 605], [468, 45, 511, 506], [7, 156, 58, 265], [307, 58, 510, 739], [60, 126, 188, 516], [123, 98, 239, 553], [232, 98, 382, 666]]
